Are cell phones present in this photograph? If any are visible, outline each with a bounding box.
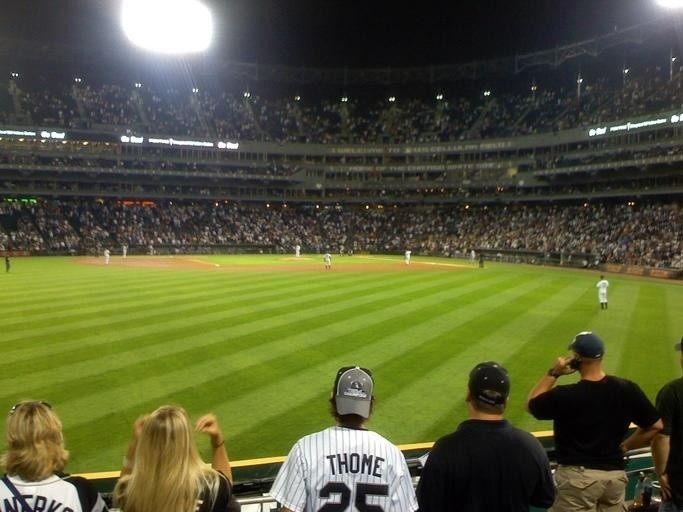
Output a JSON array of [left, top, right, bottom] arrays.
[[570, 358, 583, 370]]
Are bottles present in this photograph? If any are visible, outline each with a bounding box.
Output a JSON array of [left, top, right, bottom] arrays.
[[633, 472, 646, 508]]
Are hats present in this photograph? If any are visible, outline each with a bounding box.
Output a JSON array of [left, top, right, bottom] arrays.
[[334, 367, 374, 418], [566, 332, 605, 357], [469, 361, 510, 405]]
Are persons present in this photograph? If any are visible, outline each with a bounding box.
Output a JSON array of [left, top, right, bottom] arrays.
[[595, 275, 609, 309], [110, 403, 238, 511], [632, 468, 653, 500], [0, 397, 111, 512], [269, 365, 421, 511], [0, 66, 682, 272], [651, 337, 683, 512], [414, 361, 555, 512], [524, 330, 663, 512]]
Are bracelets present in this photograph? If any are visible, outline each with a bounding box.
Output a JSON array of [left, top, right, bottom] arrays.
[[210, 439, 224, 448], [121, 456, 133, 470], [619, 442, 629, 452], [547, 368, 560, 378]]
